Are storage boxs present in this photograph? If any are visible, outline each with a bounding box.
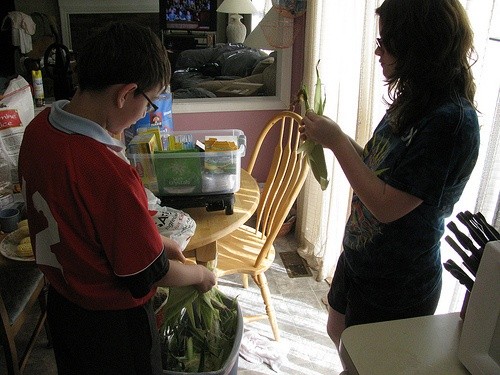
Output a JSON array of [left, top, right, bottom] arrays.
[[162, 296, 244, 375], [124, 128, 247, 196]]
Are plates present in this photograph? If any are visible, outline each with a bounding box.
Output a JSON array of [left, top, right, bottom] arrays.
[[0, 230, 36, 262]]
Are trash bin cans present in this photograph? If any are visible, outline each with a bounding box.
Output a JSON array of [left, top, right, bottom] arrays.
[[152, 292, 244, 375]]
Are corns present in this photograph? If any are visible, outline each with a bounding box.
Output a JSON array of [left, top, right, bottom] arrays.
[[12, 218, 34, 257], [300, 88, 329, 190]]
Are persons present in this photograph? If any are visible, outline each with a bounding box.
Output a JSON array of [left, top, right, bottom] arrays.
[[299, 0, 482, 375], [18, 21, 216, 375]]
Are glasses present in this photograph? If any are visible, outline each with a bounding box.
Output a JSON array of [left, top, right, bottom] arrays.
[[140, 90, 158, 114], [375, 37, 386, 47]]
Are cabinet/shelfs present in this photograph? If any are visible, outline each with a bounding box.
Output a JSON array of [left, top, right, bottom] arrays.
[[167, 33, 213, 48]]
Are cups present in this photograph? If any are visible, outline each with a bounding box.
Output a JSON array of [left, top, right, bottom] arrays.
[[0, 208, 20, 233]]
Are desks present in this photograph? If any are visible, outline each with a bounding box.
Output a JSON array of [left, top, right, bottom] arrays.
[[0, 167, 260, 347], [338, 313, 464, 375]]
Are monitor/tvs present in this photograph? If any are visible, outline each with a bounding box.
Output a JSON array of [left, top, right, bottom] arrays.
[[159, 0, 217, 32]]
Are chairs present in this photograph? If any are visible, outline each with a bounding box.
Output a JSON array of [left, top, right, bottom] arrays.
[[180, 110, 309, 340], [0, 267, 52, 375]]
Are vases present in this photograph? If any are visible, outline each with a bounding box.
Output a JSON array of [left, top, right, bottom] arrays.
[[277, 220, 295, 236]]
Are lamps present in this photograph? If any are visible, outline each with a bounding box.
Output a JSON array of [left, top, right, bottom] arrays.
[[217, 0, 251, 44]]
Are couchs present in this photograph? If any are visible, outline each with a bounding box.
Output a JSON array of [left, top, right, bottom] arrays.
[[172, 42, 276, 99]]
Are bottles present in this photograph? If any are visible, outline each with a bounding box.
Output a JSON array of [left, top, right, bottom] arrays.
[[31, 70, 45, 107]]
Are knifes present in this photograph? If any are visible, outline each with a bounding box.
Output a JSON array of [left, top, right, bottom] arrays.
[[444, 211, 499, 294]]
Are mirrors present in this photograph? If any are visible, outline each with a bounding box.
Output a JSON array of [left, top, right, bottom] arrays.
[[159, 0, 284, 103]]
